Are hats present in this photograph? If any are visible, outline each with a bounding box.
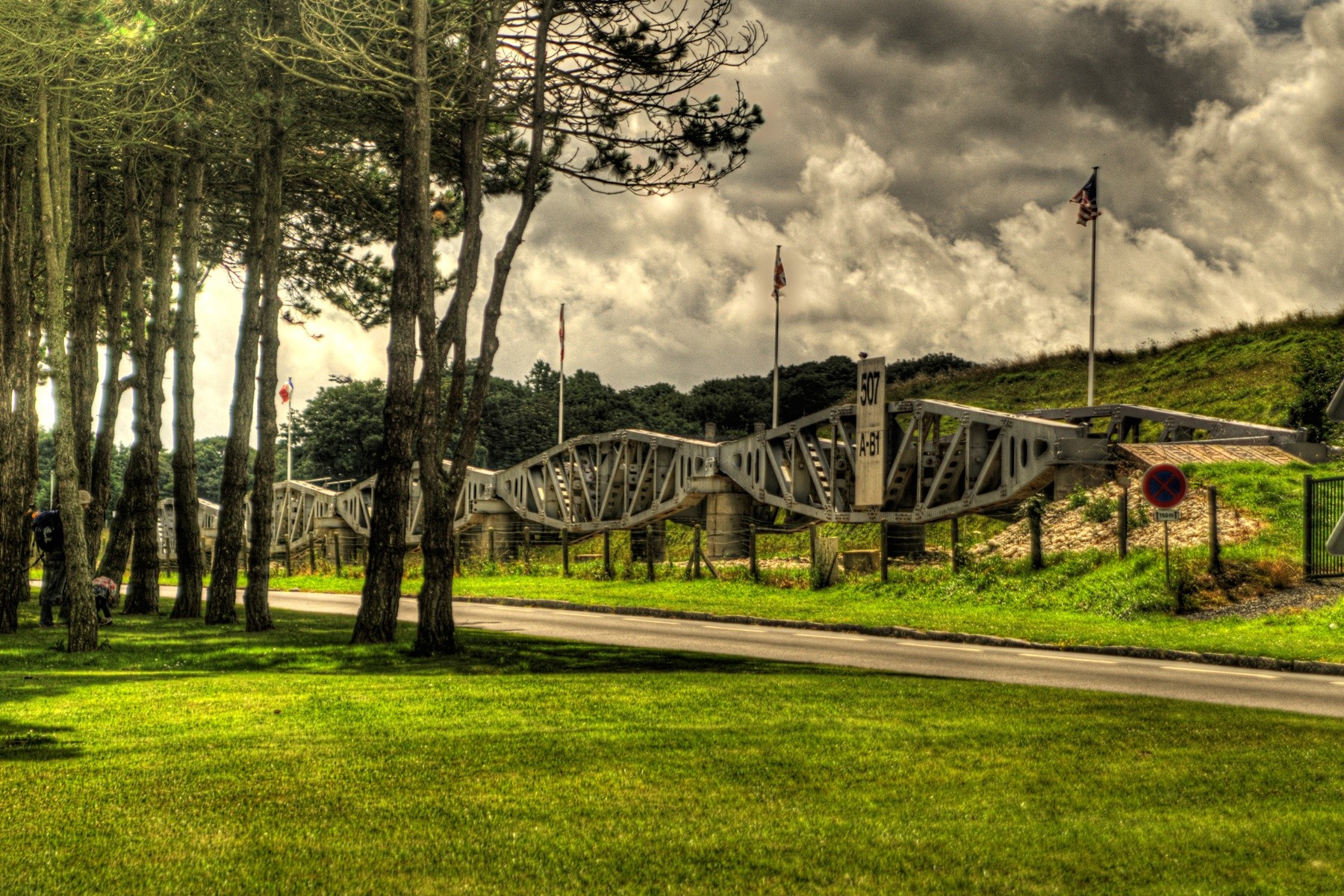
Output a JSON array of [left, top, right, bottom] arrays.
[[77, 490, 94, 504]]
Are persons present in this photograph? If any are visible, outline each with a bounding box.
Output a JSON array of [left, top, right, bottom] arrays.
[[33, 491, 117, 628]]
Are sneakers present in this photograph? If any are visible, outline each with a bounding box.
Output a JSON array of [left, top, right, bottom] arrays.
[[97, 617, 112, 625], [38, 622, 58, 628]]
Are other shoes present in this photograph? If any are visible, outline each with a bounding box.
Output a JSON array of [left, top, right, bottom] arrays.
[[57, 621, 69, 628]]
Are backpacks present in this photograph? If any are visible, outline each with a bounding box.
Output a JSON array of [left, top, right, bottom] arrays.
[[32, 510, 63, 553]]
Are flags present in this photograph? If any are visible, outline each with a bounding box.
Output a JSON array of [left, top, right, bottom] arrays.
[[279, 379, 293, 404], [559, 305, 565, 361], [771, 246, 786, 302], [1070, 172, 1101, 226]]
